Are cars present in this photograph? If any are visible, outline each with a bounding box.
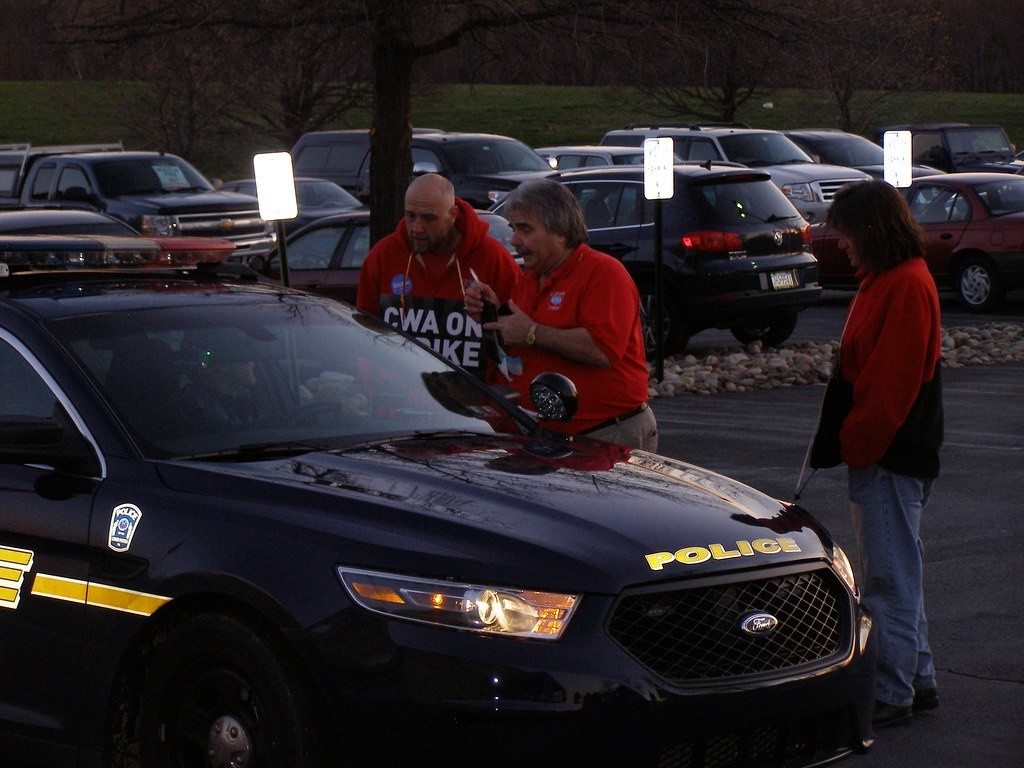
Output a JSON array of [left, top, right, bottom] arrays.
[[2, 126, 1024, 310], [488, 164, 819, 350], [2, 207, 164, 270], [854, 174, 1024, 308], [216, 175, 375, 224], [1, 267, 878, 768]]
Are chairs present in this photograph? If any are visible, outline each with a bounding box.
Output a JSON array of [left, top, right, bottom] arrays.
[[108, 337, 184, 439], [585, 199, 609, 229], [926, 197, 949, 223]]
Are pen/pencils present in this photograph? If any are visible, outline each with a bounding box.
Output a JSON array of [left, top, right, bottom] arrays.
[[467, 265, 485, 294]]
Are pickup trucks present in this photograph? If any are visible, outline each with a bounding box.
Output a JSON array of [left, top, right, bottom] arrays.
[[1, 142, 280, 260]]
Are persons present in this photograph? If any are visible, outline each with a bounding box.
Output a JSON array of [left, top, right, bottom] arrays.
[[355, 172, 524, 388], [463, 178, 658, 454], [809, 179, 945, 730], [183, 361, 355, 436]]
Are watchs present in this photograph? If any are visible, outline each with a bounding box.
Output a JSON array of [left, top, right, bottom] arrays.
[[526, 323, 539, 344]]
